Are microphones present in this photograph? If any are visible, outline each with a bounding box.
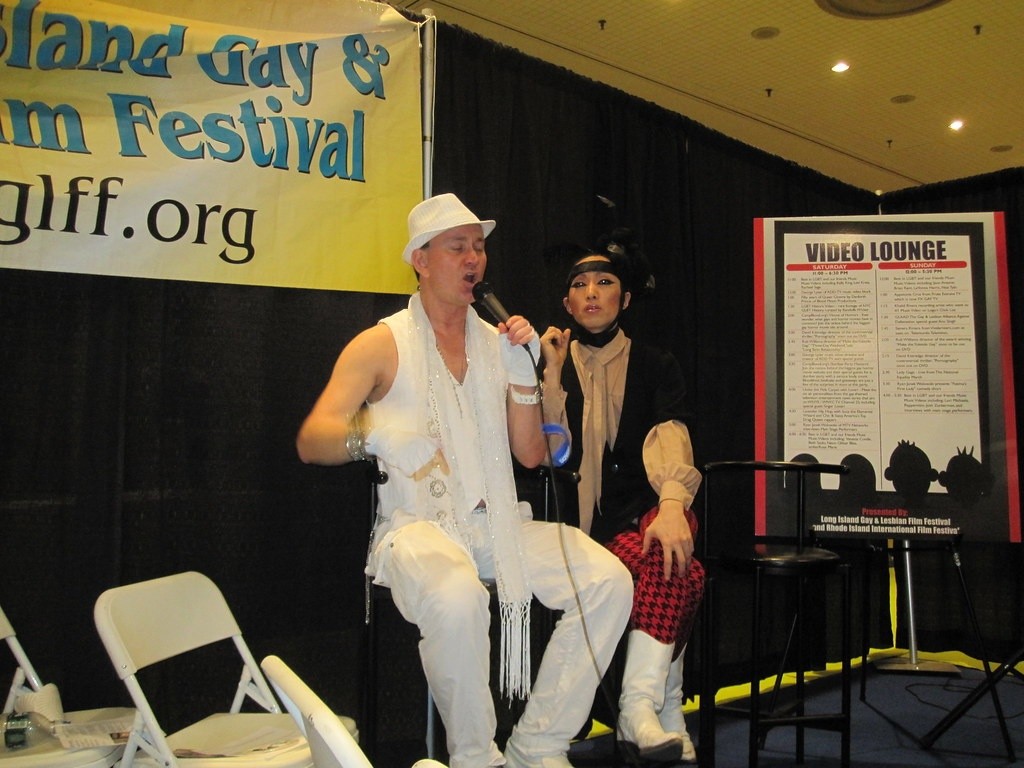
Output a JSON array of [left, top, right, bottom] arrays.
[[473, 281, 531, 351]]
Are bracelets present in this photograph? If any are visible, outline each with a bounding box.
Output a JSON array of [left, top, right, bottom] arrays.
[[345, 429, 367, 462]]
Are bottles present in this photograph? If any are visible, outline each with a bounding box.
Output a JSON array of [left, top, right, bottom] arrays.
[[0, 712, 70, 759]]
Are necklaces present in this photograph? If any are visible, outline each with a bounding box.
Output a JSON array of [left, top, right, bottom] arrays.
[[434, 316, 467, 387]]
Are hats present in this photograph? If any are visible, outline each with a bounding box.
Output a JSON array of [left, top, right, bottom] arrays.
[[400, 192, 497, 267]]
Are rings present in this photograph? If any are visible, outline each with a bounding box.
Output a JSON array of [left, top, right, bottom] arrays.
[[431, 459, 444, 468]]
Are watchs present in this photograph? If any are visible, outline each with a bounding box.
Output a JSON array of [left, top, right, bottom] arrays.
[[509, 379, 544, 404]]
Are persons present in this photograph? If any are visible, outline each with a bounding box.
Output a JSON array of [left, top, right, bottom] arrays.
[[539, 251, 703, 761], [296, 193, 634, 768]]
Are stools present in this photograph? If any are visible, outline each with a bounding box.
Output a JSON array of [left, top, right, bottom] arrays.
[[699, 461, 851, 768], [366, 462, 581, 768]]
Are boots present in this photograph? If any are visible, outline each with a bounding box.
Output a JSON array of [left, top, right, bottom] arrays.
[[662, 644, 697, 763], [616, 629, 684, 765]]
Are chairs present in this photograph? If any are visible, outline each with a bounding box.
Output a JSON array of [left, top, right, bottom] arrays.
[[261, 655, 373, 768], [0, 607, 135, 768], [94, 571, 359, 768]]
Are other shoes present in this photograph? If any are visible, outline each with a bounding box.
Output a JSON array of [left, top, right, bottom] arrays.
[[502, 744, 575, 768]]
